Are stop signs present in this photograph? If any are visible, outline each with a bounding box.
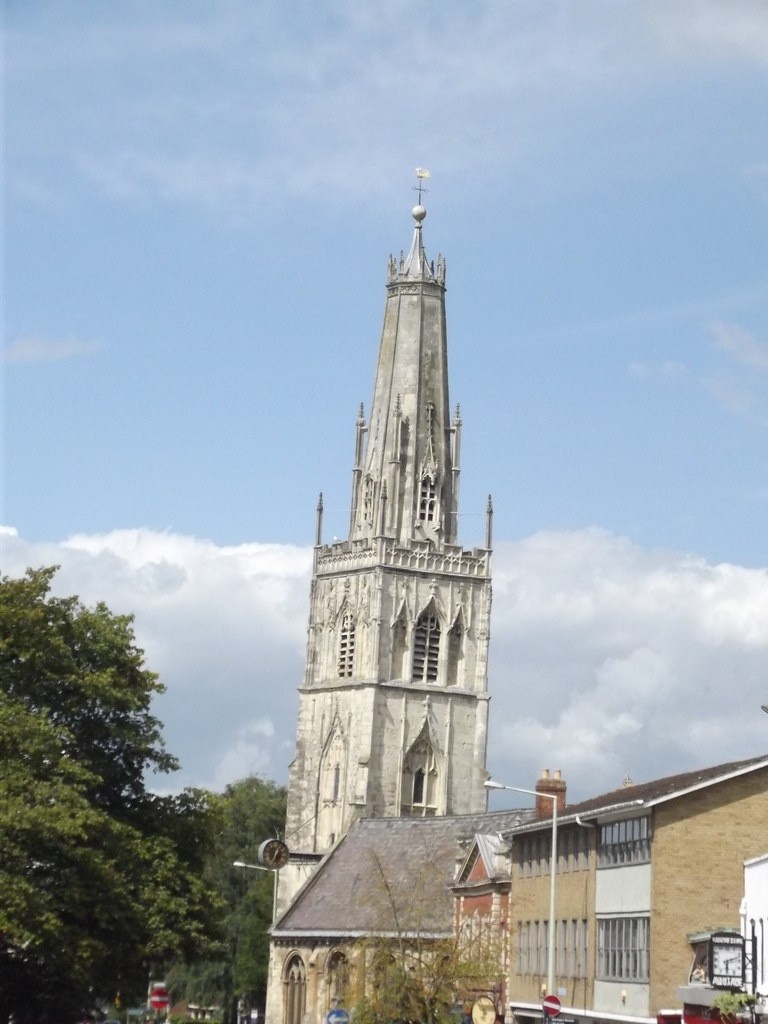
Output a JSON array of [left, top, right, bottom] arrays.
[[149, 988, 169, 1010], [542, 995, 561, 1018]]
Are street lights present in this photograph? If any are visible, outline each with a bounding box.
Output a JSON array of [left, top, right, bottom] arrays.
[[233, 861, 277, 925], [482, 781, 557, 1024]]
